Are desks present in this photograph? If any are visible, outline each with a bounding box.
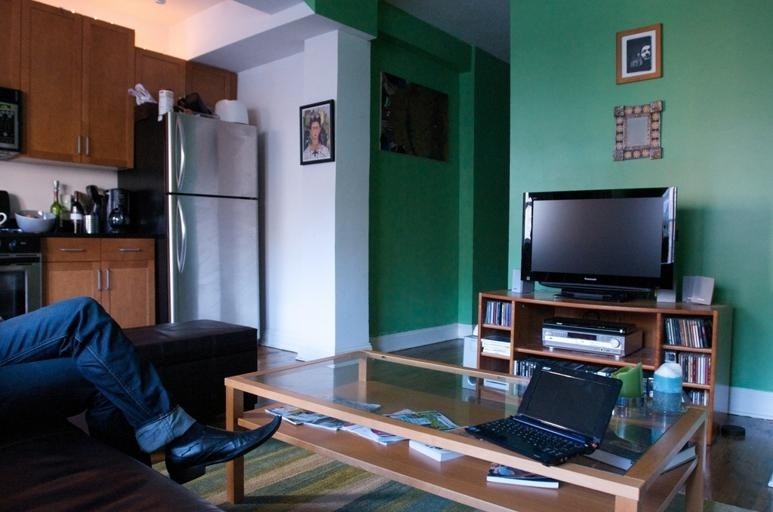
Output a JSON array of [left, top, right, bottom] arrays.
[[224, 350, 710, 512]]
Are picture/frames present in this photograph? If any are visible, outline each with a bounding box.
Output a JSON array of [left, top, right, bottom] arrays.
[[615, 23, 663, 84], [300, 100, 334, 165], [613, 100, 663, 162]]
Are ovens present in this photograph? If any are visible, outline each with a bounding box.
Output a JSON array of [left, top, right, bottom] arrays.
[[0, 252, 42, 322]]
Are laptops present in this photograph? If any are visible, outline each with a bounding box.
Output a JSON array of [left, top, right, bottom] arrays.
[[464, 361, 623, 467]]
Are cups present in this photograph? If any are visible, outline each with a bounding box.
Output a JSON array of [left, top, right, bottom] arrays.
[[83, 215, 99, 235]]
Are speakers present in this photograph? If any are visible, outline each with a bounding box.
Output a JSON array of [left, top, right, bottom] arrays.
[[462, 335, 478, 392]]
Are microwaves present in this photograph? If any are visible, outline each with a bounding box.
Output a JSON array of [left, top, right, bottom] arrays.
[[1, 86, 23, 161]]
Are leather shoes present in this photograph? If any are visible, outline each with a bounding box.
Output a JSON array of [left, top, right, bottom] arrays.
[[165, 415, 281, 485]]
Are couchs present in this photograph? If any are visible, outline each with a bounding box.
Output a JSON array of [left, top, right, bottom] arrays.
[[0, 433, 222, 512]]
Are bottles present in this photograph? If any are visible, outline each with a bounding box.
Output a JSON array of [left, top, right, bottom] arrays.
[[612, 362, 647, 419], [651, 361, 683, 414], [70, 197, 82, 234], [50, 179, 65, 232]]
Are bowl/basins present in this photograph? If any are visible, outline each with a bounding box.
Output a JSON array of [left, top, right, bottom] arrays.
[[14, 209, 55, 232]]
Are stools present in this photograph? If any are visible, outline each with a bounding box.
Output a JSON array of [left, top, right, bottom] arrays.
[[124, 326, 178, 395], [156, 319, 257, 422]]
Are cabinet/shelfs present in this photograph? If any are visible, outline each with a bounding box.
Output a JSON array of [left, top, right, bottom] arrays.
[[43, 238, 155, 328], [136, 48, 237, 117], [10, 0, 135, 169], [478, 289, 732, 446]]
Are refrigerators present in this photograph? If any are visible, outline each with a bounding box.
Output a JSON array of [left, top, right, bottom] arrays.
[[115, 111, 259, 342]]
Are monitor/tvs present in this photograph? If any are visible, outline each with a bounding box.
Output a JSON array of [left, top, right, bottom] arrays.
[[521, 187, 678, 302]]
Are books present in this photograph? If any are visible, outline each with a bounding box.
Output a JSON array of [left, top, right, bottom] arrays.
[[485, 463, 560, 489], [265, 397, 484, 462], [583, 423, 697, 475], [480, 301, 511, 356], [514, 316, 712, 418]]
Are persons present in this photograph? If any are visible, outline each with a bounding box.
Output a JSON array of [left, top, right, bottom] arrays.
[[639, 41, 651, 71], [0, 297, 282, 484], [303, 117, 330, 162]]
